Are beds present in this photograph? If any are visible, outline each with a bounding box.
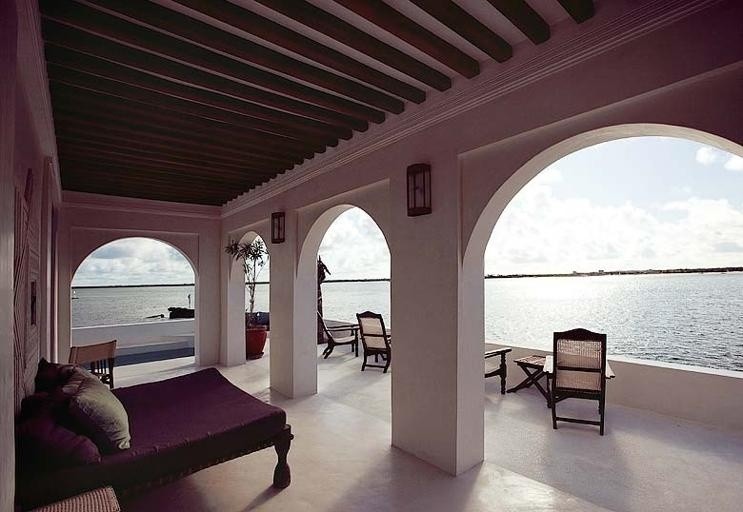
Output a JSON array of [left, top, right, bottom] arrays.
[[25, 366, 294, 503]]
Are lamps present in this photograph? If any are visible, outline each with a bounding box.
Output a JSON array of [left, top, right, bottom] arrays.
[[271, 212, 286, 244], [408, 164, 433, 216]]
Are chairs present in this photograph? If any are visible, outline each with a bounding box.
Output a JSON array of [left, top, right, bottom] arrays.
[[485, 328, 613, 438], [318, 310, 390, 373], [69, 340, 118, 391]]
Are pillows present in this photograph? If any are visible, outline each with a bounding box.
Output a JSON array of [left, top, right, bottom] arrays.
[[26, 359, 131, 465]]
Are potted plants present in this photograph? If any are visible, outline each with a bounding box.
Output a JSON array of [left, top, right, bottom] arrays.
[[225, 237, 269, 360]]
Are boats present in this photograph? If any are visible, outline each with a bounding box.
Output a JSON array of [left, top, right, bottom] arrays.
[[72, 290, 79, 299]]
